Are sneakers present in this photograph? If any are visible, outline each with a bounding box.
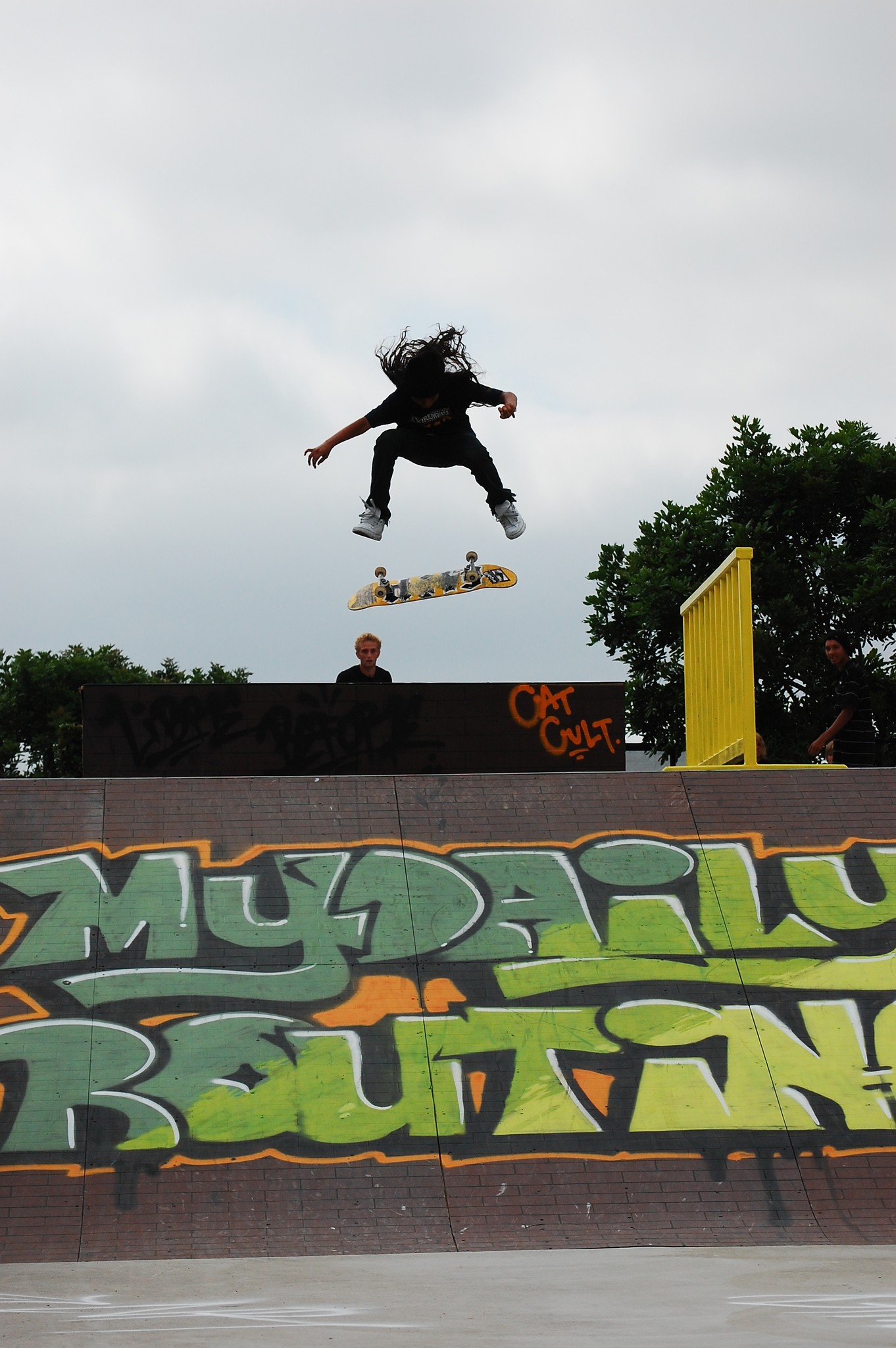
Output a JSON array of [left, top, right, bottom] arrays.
[[494, 501, 525, 539], [352, 496, 385, 541]]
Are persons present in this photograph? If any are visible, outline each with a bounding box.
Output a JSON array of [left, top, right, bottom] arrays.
[[738, 733, 769, 765], [808, 632, 879, 768], [336, 634, 393, 683], [304, 323, 526, 540]]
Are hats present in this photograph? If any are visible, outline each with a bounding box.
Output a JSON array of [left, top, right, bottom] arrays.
[[401, 350, 445, 399], [823, 630, 853, 658]]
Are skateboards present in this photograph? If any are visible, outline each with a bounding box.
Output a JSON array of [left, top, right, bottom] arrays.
[[347, 551, 518, 612]]
